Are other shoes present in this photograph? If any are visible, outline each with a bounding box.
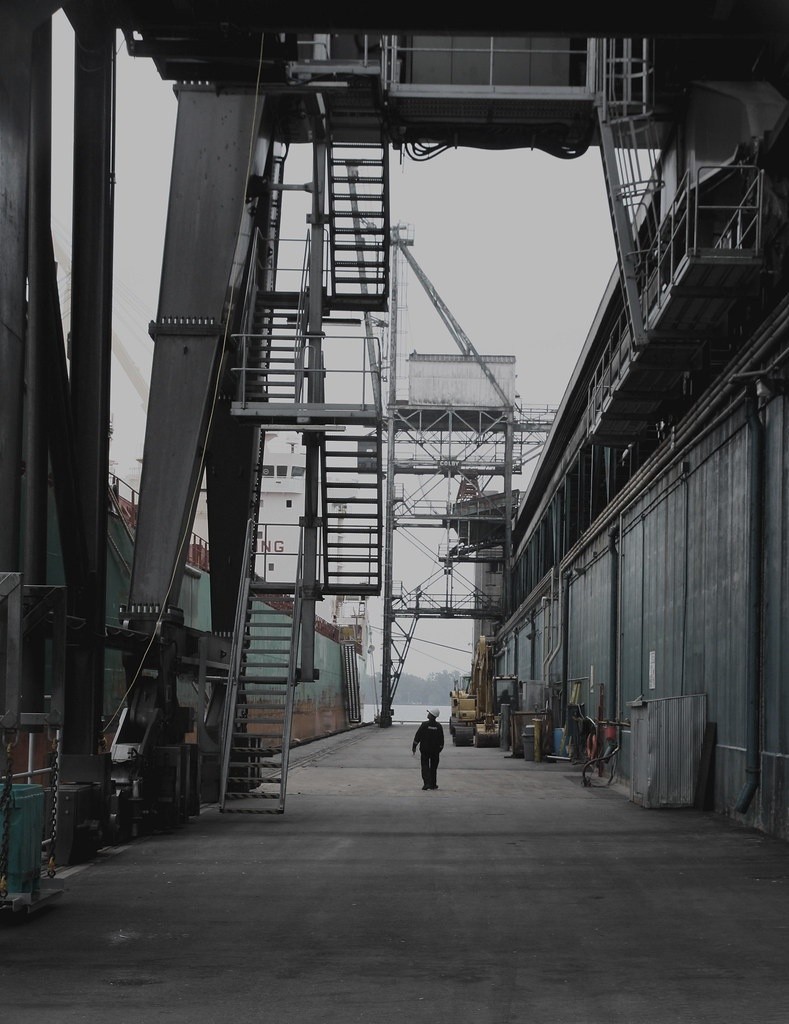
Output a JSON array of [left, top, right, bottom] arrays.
[[429, 784, 438, 789], [422, 784, 430, 790]]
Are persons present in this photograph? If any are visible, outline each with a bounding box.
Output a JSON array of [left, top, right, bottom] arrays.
[[411, 707, 445, 790]]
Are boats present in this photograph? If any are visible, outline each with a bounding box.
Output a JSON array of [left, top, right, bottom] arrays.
[[14, 427, 378, 859]]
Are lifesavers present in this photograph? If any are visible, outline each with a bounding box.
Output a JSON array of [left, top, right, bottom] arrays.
[[586, 732, 596, 759]]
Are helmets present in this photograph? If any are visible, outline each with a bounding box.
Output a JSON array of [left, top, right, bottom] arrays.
[[426, 707, 440, 717]]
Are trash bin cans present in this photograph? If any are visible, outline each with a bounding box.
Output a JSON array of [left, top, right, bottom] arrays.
[[521, 725, 536, 761]]
